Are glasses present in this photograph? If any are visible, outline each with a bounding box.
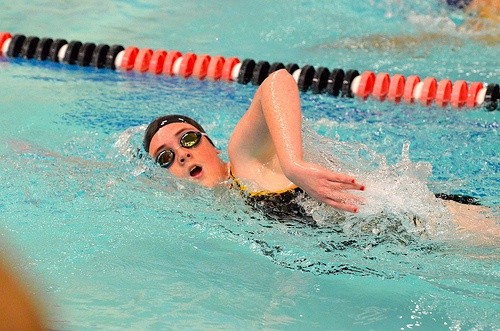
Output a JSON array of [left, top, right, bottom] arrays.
[[152, 130, 207, 167]]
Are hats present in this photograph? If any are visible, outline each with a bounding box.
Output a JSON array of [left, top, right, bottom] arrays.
[[143, 115, 214, 155]]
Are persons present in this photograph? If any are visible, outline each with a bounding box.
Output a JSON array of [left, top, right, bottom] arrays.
[[7, 66, 500, 262], [304, 0, 500, 54]]
